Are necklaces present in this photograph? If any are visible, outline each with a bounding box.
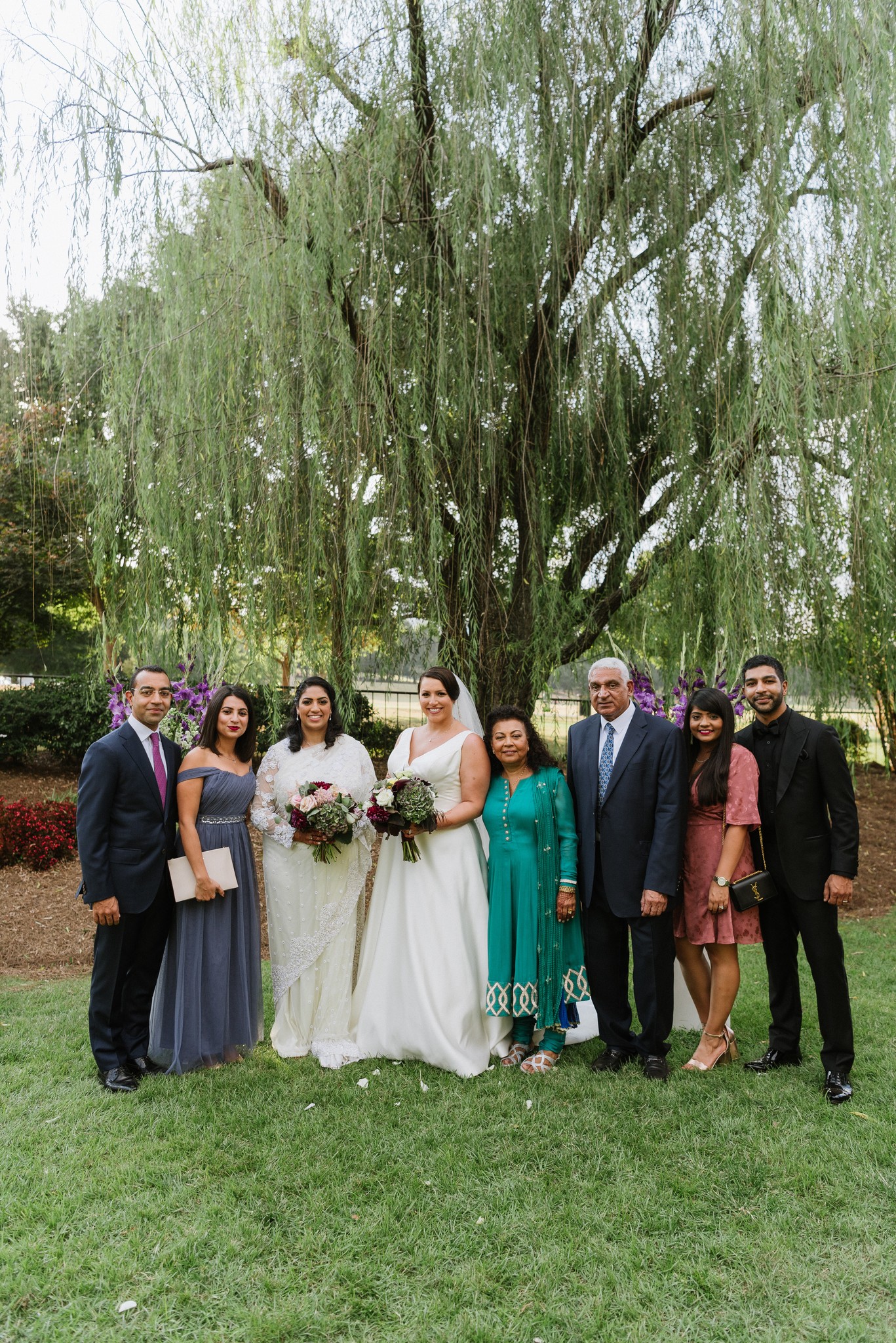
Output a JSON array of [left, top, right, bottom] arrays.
[[302, 735, 326, 755], [426, 718, 456, 741], [222, 753, 238, 763], [501, 763, 528, 788], [695, 758, 709, 762]]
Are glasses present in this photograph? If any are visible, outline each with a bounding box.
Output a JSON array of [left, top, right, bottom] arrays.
[[129, 688, 173, 697]]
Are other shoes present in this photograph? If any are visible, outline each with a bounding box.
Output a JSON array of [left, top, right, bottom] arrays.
[[206, 1064, 223, 1071], [230, 1052, 244, 1065]]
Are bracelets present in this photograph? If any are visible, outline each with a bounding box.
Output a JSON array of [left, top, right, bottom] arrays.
[[559, 886, 576, 893], [560, 879, 577, 885]]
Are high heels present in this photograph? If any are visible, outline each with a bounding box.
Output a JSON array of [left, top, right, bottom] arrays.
[[702, 1021, 741, 1060], [683, 1030, 730, 1071]]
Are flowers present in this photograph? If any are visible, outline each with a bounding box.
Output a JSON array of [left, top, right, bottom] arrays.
[[364, 767, 438, 863], [285, 780, 364, 864]]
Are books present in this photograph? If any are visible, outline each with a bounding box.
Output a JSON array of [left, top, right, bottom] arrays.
[[168, 847, 238, 902]]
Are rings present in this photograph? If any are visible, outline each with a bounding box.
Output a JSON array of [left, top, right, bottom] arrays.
[[719, 905, 724, 909], [408, 828, 412, 835], [568, 909, 572, 914], [311, 836, 313, 840], [843, 901, 848, 902]]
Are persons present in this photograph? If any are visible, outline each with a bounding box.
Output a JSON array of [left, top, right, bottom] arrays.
[[482, 704, 590, 1075], [148, 685, 265, 1076], [75, 665, 181, 1092], [351, 667, 600, 1079], [567, 657, 690, 1082], [250, 677, 379, 1069], [672, 688, 764, 1071], [733, 655, 859, 1104]]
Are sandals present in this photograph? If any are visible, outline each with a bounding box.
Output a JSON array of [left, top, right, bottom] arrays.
[[500, 1038, 535, 1068], [520, 1049, 564, 1076]]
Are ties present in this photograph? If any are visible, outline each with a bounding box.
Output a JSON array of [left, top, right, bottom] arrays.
[[595, 722, 617, 834], [150, 732, 168, 810]]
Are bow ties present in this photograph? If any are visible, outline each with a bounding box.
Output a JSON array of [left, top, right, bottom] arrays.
[[755, 719, 779, 739]]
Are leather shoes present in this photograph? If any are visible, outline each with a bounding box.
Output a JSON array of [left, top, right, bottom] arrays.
[[97, 1063, 141, 1095], [125, 1055, 173, 1081], [643, 1055, 669, 1083], [590, 1048, 637, 1073], [744, 1047, 802, 1074], [823, 1070, 852, 1104]]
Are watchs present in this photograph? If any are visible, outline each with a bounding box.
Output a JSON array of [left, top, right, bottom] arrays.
[[713, 875, 731, 887]]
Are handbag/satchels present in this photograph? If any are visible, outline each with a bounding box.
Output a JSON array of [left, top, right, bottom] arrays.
[[728, 869, 778, 912]]
